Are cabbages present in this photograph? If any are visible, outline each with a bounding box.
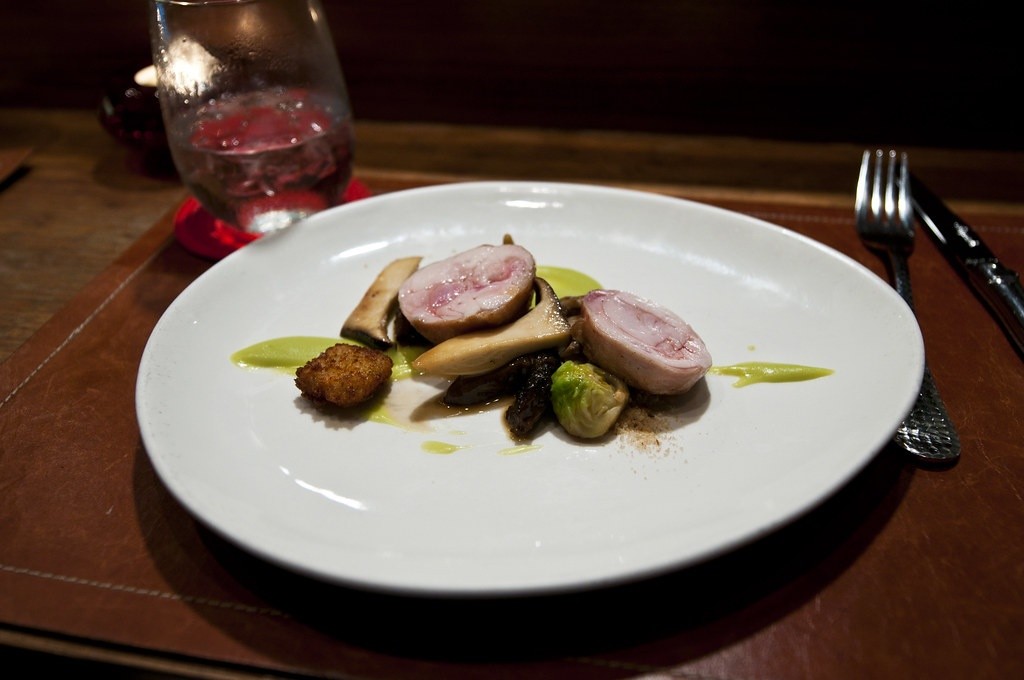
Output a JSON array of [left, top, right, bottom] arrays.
[[551, 360, 628, 439]]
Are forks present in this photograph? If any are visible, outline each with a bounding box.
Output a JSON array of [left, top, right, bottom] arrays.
[[853, 145, 961, 465]]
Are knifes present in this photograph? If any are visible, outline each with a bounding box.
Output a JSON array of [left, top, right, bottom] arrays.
[[909, 176, 1022, 354]]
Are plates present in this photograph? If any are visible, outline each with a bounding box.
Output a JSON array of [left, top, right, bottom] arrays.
[[134, 181, 924, 597]]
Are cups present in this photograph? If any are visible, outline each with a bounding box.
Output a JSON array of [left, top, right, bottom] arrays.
[[145, 1, 358, 238]]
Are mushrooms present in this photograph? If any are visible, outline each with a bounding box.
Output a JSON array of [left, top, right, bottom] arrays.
[[341, 254, 572, 377]]
